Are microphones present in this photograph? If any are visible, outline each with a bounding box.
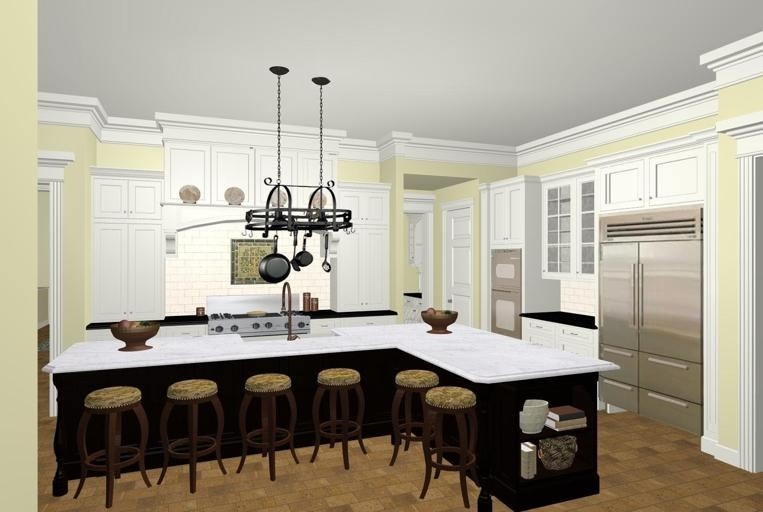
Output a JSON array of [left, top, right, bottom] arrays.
[[73, 385, 152, 509], [234, 371, 299, 482], [309, 366, 368, 474], [388, 369, 439, 469], [418, 384, 480, 508], [155, 378, 226, 494]]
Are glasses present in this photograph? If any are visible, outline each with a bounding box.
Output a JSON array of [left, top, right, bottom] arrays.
[[242, 64, 355, 283]]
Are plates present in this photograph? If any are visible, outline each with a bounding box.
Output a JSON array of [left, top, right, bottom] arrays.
[[594, 148, 702, 206], [333, 180, 392, 227], [252, 144, 338, 210], [515, 380, 589, 491], [162, 137, 256, 208], [487, 174, 540, 248], [403, 296, 422, 323], [329, 227, 390, 312], [89, 217, 166, 322], [521, 316, 606, 413], [88, 165, 164, 222]]
